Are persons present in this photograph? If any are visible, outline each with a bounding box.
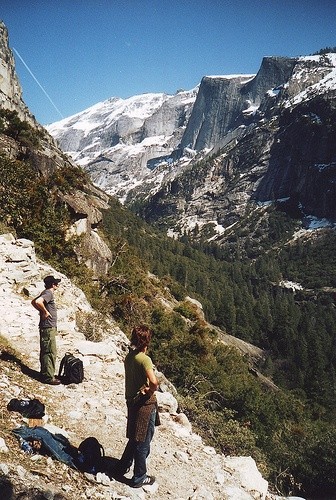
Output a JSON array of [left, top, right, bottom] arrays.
[[31, 275, 62, 385], [116, 326, 161, 487]]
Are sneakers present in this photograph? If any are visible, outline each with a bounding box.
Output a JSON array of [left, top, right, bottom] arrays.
[[134, 475, 155, 488]]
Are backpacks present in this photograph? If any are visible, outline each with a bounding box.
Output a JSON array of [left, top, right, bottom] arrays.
[[77, 436, 105, 476], [58, 352, 84, 384]]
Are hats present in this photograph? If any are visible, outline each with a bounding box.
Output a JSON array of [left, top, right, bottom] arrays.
[[44, 276, 61, 283]]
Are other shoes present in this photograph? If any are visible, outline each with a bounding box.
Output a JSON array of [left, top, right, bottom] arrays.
[[47, 379, 61, 385]]
